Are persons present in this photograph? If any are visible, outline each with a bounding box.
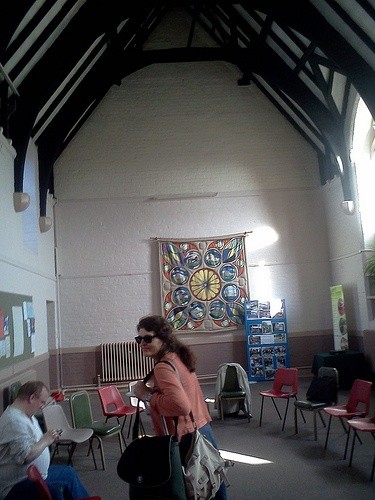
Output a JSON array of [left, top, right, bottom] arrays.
[[134, 315, 228, 500], [0, 380, 103, 500]]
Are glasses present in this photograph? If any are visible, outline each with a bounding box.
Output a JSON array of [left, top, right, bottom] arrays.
[[134, 335, 157, 344]]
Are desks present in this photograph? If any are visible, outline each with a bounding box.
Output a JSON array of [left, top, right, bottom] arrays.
[[311, 353, 368, 391]]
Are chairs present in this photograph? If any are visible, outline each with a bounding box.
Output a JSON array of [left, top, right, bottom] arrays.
[[294, 375, 348, 442], [127, 380, 150, 438], [97, 385, 146, 450], [214, 362, 253, 423], [259, 367, 306, 431], [344, 415, 375, 482], [10, 381, 123, 471], [322, 379, 375, 459]]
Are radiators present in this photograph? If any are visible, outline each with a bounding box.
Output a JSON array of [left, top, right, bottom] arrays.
[[100, 342, 155, 382]]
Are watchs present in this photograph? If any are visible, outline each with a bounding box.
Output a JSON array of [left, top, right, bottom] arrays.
[[146, 390, 155, 403]]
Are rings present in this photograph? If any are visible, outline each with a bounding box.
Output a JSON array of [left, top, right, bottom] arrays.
[[52, 433, 55, 436]]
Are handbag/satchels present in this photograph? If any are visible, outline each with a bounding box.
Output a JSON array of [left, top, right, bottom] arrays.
[[119, 435, 189, 500], [180, 430, 234, 499], [305, 376, 340, 405]]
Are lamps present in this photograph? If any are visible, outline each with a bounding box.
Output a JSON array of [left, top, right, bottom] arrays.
[[236, 74, 251, 88]]
